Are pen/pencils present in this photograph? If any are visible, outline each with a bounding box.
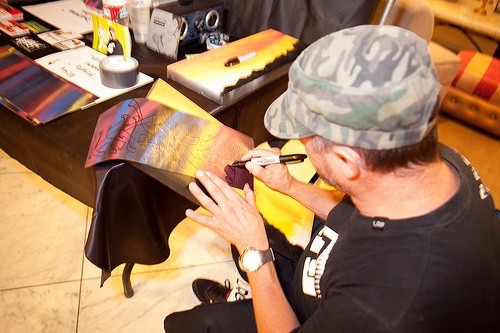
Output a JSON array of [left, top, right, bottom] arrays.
[[230, 154, 307, 168], [225, 51, 257, 66]]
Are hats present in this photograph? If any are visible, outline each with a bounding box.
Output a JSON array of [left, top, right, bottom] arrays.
[[263, 24, 440, 150]]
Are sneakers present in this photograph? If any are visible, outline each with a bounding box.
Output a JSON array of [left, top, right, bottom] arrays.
[[192, 279, 238, 305]]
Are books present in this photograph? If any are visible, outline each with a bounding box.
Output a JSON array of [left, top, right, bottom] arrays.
[[0, 0, 85, 57]]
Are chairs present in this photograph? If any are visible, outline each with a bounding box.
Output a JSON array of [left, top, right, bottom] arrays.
[[386, 2, 461, 112]]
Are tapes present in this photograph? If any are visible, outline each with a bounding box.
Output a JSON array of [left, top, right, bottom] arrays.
[[207, 35, 227, 50], [99, 55, 140, 89]]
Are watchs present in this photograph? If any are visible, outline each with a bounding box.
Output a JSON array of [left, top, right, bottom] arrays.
[[238, 247, 275, 273]]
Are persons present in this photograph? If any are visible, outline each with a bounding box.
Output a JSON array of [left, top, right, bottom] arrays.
[[163, 24, 500, 333], [106, 27, 124, 56], [152, 14, 182, 58]]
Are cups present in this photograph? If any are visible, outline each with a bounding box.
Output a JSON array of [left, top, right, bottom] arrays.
[[127, 0, 151, 44]]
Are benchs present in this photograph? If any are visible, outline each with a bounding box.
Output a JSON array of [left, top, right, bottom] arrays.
[[442, 48, 500, 136]]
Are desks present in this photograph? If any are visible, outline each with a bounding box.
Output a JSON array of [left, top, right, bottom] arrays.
[[392, 0, 500, 60], [0, 0, 319, 298]]
